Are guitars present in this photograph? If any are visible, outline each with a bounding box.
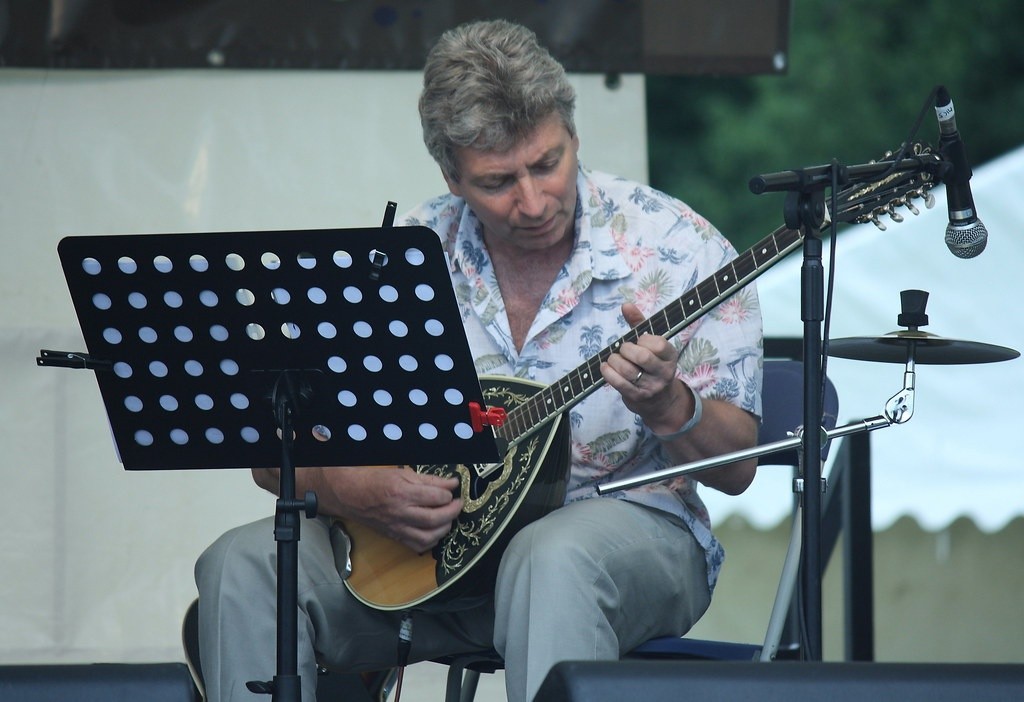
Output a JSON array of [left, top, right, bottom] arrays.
[[327, 140, 941, 620]]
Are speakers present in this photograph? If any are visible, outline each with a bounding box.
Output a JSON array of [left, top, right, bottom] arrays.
[[530, 660, 1022, 701]]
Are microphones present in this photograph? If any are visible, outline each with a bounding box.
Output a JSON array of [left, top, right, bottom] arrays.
[[934, 86, 988, 259]]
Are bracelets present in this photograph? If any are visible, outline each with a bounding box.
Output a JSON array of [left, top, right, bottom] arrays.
[[648, 378, 702, 442]]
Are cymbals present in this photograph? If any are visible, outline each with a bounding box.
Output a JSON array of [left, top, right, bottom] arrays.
[[822, 325, 1024, 367]]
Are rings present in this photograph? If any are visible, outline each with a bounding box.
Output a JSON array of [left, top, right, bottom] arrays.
[[631, 369, 643, 384]]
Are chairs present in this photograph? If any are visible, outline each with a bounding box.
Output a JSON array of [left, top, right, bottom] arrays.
[[312, 357, 841, 702]]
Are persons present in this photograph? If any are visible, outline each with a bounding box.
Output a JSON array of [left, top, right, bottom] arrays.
[[195, 21, 763, 702]]
